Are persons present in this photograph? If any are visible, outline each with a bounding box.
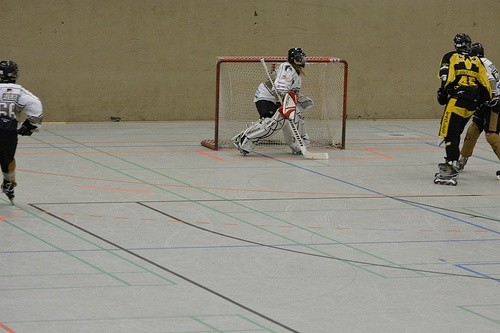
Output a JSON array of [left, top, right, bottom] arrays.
[[0, 60, 43, 205], [434, 33, 492, 185], [233, 47, 313, 156], [456, 44, 500, 180]]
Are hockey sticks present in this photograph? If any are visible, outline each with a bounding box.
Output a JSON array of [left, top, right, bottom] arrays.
[[260, 58, 329, 160]]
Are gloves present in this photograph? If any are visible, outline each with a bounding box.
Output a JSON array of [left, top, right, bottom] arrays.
[[437, 89, 448, 105], [18, 120, 38, 136], [486, 90, 499, 107]]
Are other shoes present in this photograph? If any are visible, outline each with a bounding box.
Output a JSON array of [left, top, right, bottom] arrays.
[[231, 135, 247, 156], [292, 144, 308, 154]]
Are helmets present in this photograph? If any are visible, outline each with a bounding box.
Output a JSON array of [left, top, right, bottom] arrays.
[[288, 47, 306, 68], [454, 32, 472, 53], [0, 60, 19, 83], [471, 42, 484, 57]]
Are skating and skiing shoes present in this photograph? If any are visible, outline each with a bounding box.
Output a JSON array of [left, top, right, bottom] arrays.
[[438, 157, 465, 173], [435, 165, 459, 185], [1, 179, 17, 206]]
